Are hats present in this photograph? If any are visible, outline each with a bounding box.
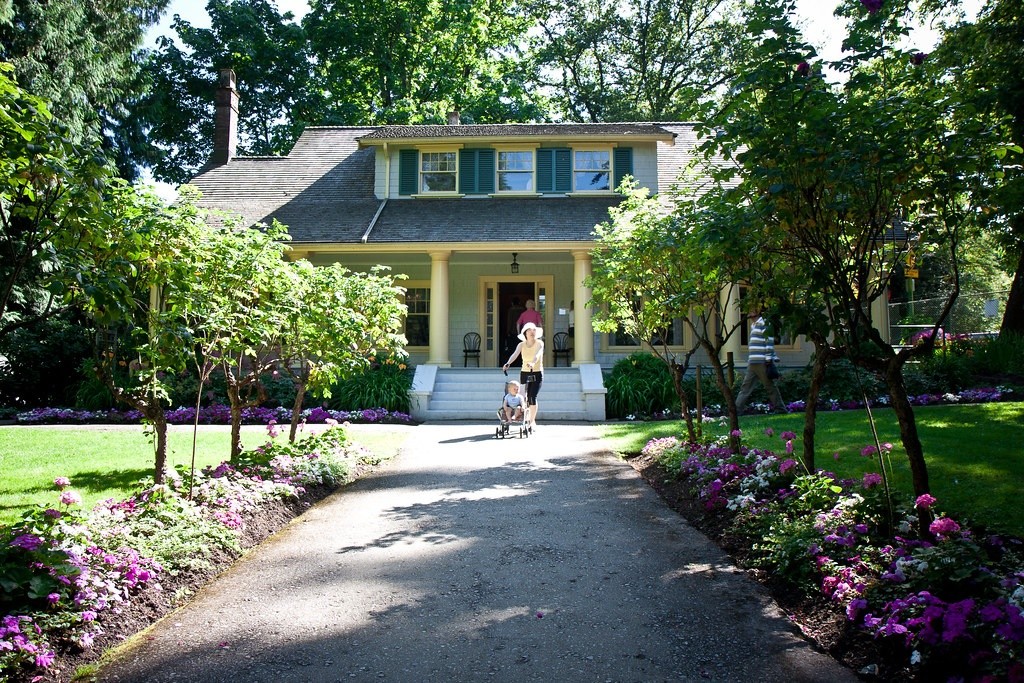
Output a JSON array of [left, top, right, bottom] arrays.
[[517, 322, 544, 342]]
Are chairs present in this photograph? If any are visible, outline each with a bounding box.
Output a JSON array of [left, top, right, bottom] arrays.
[[552, 332, 570, 367], [462, 331, 481, 368]]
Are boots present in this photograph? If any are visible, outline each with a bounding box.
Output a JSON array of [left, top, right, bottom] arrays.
[[523, 405, 537, 431]]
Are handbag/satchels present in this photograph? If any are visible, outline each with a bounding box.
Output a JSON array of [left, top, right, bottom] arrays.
[[765, 362, 779, 380]]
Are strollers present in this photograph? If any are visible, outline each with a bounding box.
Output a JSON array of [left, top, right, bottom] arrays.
[[496, 364, 532, 439]]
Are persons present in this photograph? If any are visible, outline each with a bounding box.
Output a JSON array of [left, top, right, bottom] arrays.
[[503, 381, 526, 423], [732, 308, 787, 416], [502, 322, 544, 432], [516, 299, 543, 335]]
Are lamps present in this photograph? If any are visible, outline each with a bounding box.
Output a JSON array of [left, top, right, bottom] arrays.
[[510, 253, 520, 273]]
